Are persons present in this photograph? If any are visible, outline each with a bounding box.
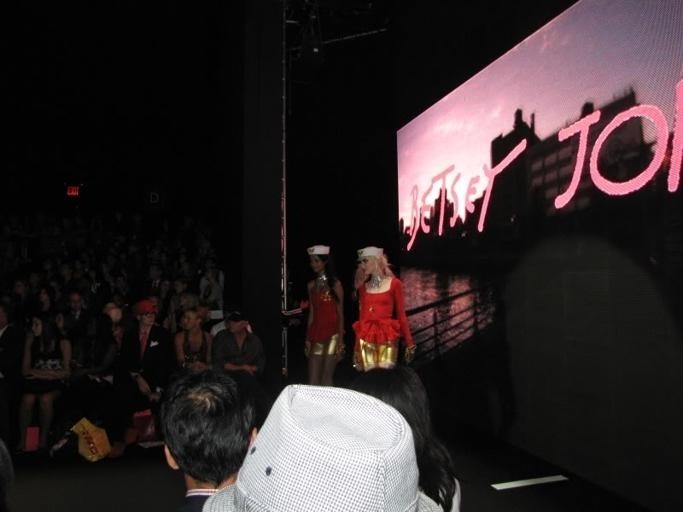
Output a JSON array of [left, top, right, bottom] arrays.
[[326, 352, 463, 512], [300, 243, 346, 388], [152, 366, 260, 510], [0, 218, 271, 470], [350, 245, 418, 396]]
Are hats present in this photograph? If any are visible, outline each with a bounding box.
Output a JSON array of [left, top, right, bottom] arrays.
[[228, 309, 247, 322], [202, 384, 444, 512], [134, 299, 157, 314], [357, 246, 384, 261], [306, 245, 330, 256]]
